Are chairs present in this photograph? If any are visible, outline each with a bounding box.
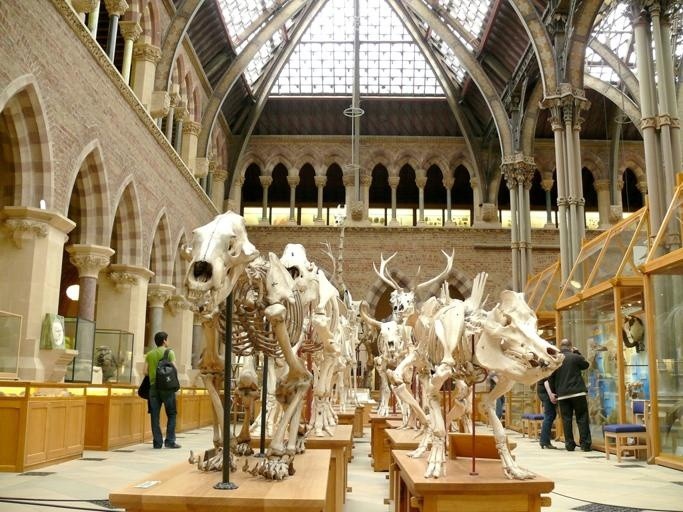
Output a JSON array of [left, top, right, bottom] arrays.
[[518, 396, 560, 441], [603, 398, 652, 463]]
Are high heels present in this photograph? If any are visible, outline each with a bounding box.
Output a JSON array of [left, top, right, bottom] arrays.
[[540, 442, 556, 449]]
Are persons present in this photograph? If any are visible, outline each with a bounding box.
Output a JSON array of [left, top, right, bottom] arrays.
[[142, 330, 181, 450], [537, 370, 557, 451], [555, 339, 593, 452], [489, 378, 505, 420]]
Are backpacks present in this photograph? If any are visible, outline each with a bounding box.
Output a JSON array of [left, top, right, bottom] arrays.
[[157, 348, 180, 391]]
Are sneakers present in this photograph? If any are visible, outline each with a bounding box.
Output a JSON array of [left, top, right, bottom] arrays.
[[171, 443, 180, 448]]
